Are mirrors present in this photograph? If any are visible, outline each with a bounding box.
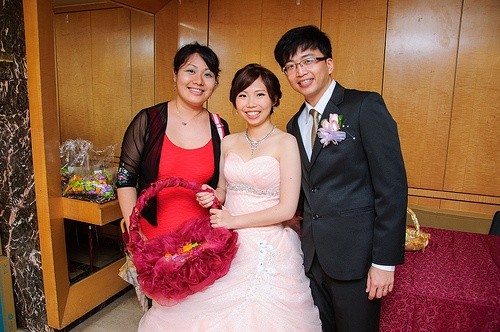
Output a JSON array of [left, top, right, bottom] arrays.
[[53, 0, 158, 286]]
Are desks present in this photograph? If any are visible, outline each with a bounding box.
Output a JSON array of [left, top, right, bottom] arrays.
[[62, 197, 123, 274], [285, 209, 500, 332]]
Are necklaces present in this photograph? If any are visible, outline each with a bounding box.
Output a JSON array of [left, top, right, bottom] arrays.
[[245, 126, 276, 155], [175, 99, 204, 126]]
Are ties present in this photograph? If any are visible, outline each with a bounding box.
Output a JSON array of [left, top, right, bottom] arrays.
[[309, 109, 319, 150]]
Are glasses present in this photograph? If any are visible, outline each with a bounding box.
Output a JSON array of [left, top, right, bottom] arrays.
[[282, 56, 330, 75]]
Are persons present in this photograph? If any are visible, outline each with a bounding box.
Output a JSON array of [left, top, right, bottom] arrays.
[[138, 63, 324, 331], [274, 24, 408, 331], [115, 42, 230, 311]]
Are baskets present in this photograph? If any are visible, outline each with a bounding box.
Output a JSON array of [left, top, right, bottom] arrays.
[[406, 208, 429, 250], [128, 177, 241, 307]]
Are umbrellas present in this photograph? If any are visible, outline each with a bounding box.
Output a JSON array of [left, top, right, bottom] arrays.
[[117, 218, 149, 313]]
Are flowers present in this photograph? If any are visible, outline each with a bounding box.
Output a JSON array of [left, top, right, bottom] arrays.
[[317, 112, 346, 148]]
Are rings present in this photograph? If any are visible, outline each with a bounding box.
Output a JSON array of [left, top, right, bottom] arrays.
[[383, 289, 388, 292]]
